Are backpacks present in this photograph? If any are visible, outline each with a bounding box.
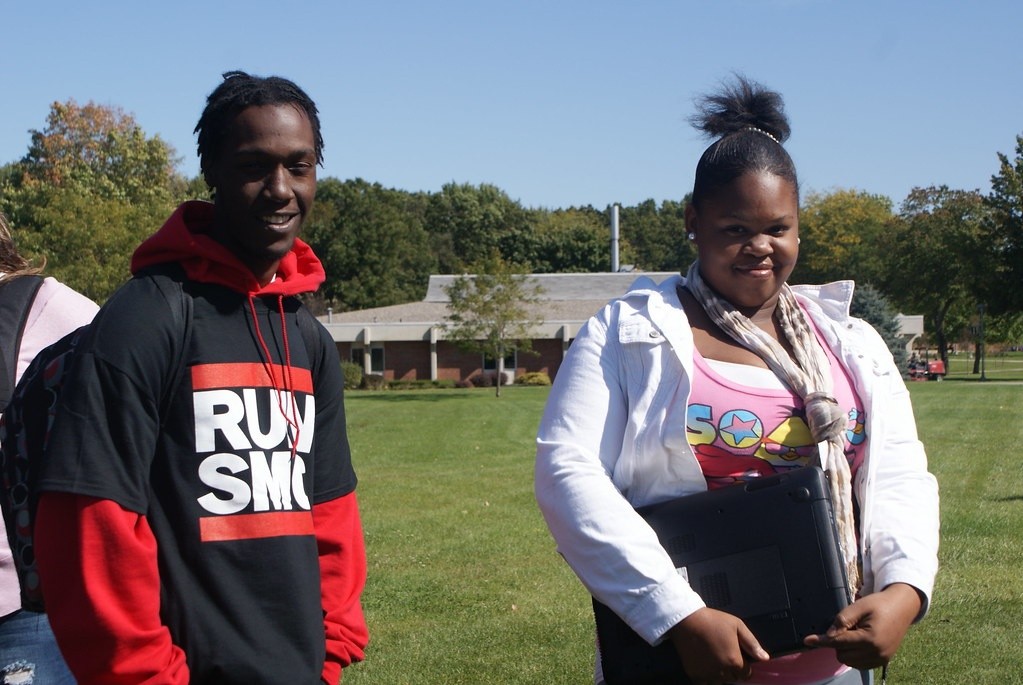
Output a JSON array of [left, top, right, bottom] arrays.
[[0, 259, 196, 616]]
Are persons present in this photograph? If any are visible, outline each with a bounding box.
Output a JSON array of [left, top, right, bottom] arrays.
[[29, 69, 369, 684], [530, 77, 944, 685], [0, 212, 107, 685]]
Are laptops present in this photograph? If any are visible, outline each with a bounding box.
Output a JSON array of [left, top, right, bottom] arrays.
[[590, 465, 852, 684]]
